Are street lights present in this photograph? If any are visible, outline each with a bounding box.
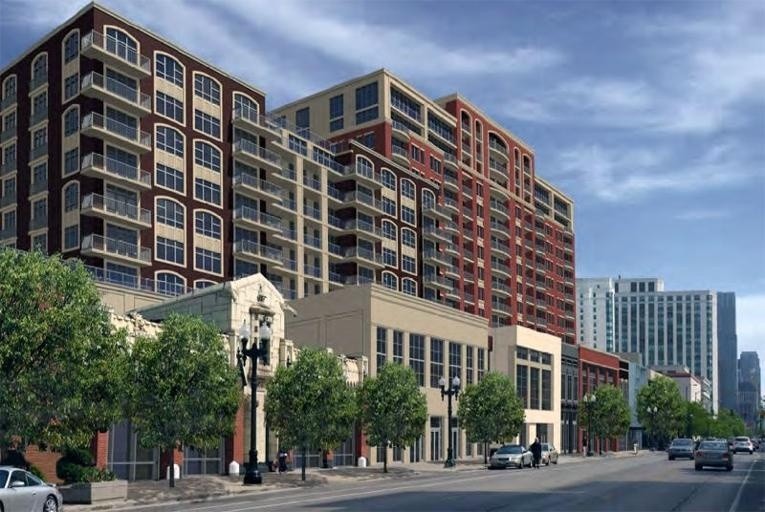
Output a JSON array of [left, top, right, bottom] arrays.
[[583, 392, 597, 457], [437, 376, 462, 468], [234, 317, 272, 486], [646, 405, 658, 451]]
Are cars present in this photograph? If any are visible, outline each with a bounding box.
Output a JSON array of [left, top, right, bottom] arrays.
[[489, 444, 534, 470], [668, 437, 697, 461], [0, 464, 64, 511], [541, 442, 559, 465], [693, 439, 734, 472], [732, 435, 762, 454]]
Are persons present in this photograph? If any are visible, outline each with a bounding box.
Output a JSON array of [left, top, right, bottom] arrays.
[[529, 438, 541, 469], [276, 447, 291, 480]]
